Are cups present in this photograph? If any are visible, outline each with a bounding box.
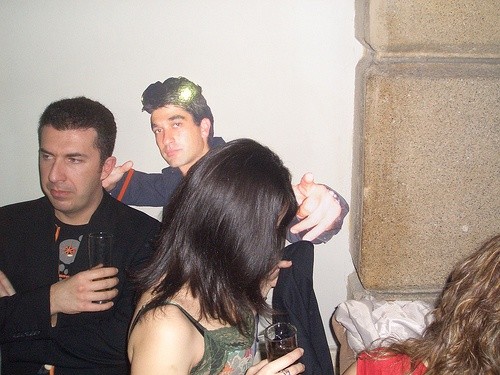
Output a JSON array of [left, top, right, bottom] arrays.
[[88, 231, 115, 305], [264, 321, 301, 375]]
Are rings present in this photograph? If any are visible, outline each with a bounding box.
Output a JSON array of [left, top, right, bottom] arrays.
[[280, 369, 290, 375]]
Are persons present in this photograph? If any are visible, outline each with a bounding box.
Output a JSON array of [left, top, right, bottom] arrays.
[[331, 236, 500, 375], [126, 138, 307, 375], [103, 77, 350, 244], [0, 96, 163, 375]]
